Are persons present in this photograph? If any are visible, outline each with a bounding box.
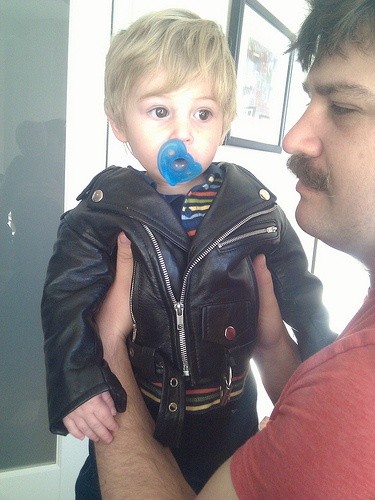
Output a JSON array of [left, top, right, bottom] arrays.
[[41, 8, 336, 500], [93, 0, 375, 499]]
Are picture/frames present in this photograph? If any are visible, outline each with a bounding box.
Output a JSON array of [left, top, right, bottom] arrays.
[[223, 0, 300, 154]]
